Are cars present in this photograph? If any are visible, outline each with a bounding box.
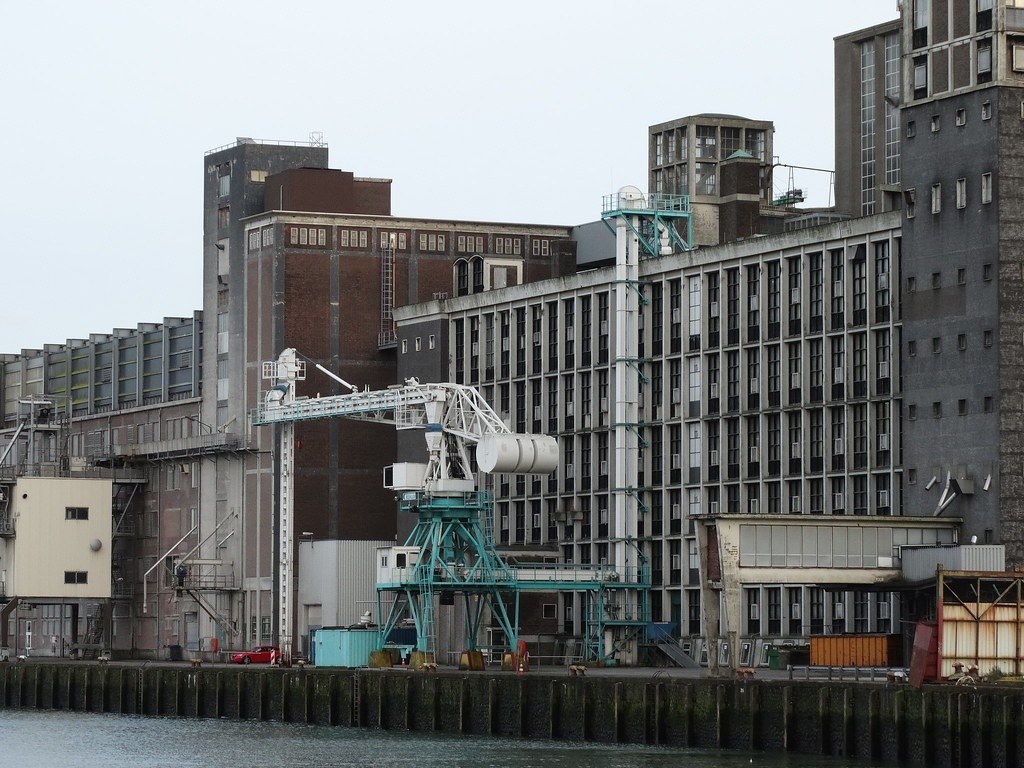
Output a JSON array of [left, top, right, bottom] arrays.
[[230, 646, 281, 665]]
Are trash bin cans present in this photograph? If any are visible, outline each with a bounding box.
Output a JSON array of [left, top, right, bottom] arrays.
[[767, 645, 809, 670], [163, 645, 181, 661], [808, 631, 902, 667]]
